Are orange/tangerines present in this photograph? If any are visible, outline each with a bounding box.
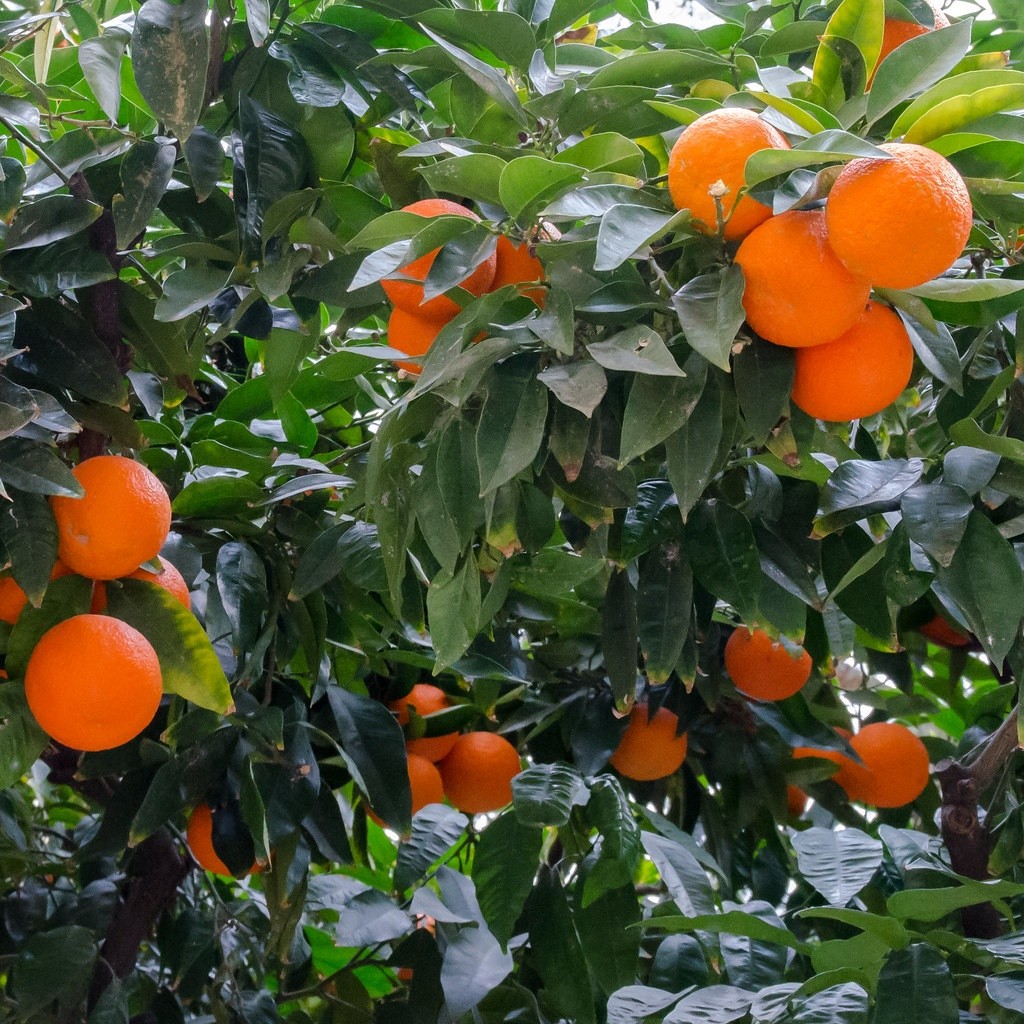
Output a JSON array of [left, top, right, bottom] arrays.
[[0, 15, 1024, 985]]
